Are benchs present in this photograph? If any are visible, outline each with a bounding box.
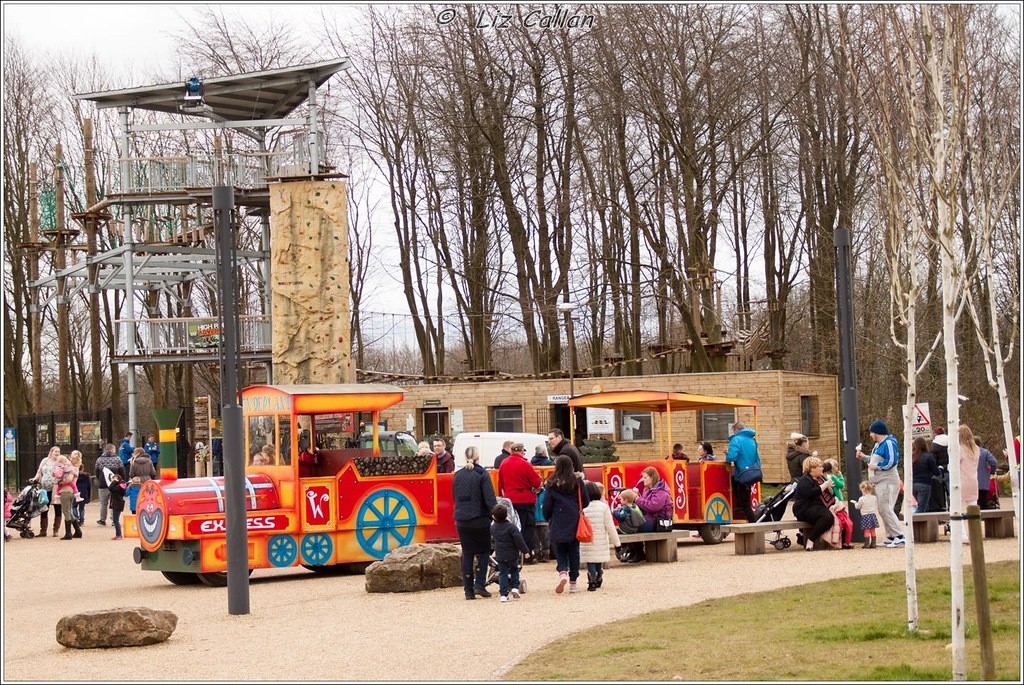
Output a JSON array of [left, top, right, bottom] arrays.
[[603, 530, 689, 568], [912, 508, 1015, 542], [719, 521, 818, 555], [312, 448, 372, 479]]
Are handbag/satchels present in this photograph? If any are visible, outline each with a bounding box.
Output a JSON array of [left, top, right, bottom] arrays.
[[734, 463, 763, 486], [35, 489, 49, 509], [657, 519, 673, 532], [575, 483, 593, 542]]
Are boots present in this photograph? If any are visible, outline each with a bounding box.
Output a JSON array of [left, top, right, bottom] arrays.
[[54, 496, 61, 504], [862, 538, 869, 549], [870, 536, 876, 547], [74, 492, 84, 502]]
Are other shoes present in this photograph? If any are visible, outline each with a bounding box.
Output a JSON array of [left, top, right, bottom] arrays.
[[805, 539, 814, 550], [524, 557, 537, 564], [629, 551, 646, 563], [4, 516, 124, 542], [596, 575, 603, 588], [511, 588, 520, 598], [620, 552, 635, 562], [587, 581, 597, 591], [474, 584, 491, 597], [535, 552, 548, 561], [555, 571, 567, 593], [843, 543, 853, 549], [569, 581, 579, 593], [500, 596, 511, 602], [465, 591, 475, 599]]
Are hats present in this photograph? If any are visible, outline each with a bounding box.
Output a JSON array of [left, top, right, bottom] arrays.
[[869, 420, 888, 435]]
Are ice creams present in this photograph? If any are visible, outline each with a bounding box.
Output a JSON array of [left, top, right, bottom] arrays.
[[855, 443, 862, 452], [812, 451, 817, 456], [633, 487, 639, 495]]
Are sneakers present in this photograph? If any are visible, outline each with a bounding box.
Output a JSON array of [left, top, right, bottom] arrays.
[[875, 536, 892, 546], [887, 536, 905, 548]]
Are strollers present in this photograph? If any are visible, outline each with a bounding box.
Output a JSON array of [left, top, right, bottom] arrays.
[[751, 478, 799, 551], [608, 487, 633, 561], [482, 497, 527, 594], [933, 465, 950, 535], [988, 467, 1000, 509], [6, 479, 49, 539]]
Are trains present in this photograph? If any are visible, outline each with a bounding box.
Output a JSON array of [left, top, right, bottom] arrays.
[[122, 384, 757, 588]]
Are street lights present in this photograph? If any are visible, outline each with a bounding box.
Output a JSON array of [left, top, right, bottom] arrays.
[[559, 304, 581, 445]]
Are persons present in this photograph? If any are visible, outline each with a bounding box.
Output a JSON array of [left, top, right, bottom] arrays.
[[542, 455, 592, 595], [451, 446, 501, 600], [432, 438, 455, 473], [624, 464, 673, 562], [610, 488, 645, 562], [530, 444, 555, 466], [546, 428, 585, 479], [244, 415, 323, 480], [498, 445, 540, 564], [26, 431, 161, 539], [533, 477, 551, 564], [494, 439, 515, 470], [698, 441, 717, 464], [665, 443, 690, 463], [578, 482, 622, 592], [488, 505, 530, 602], [725, 412, 1022, 555]]
